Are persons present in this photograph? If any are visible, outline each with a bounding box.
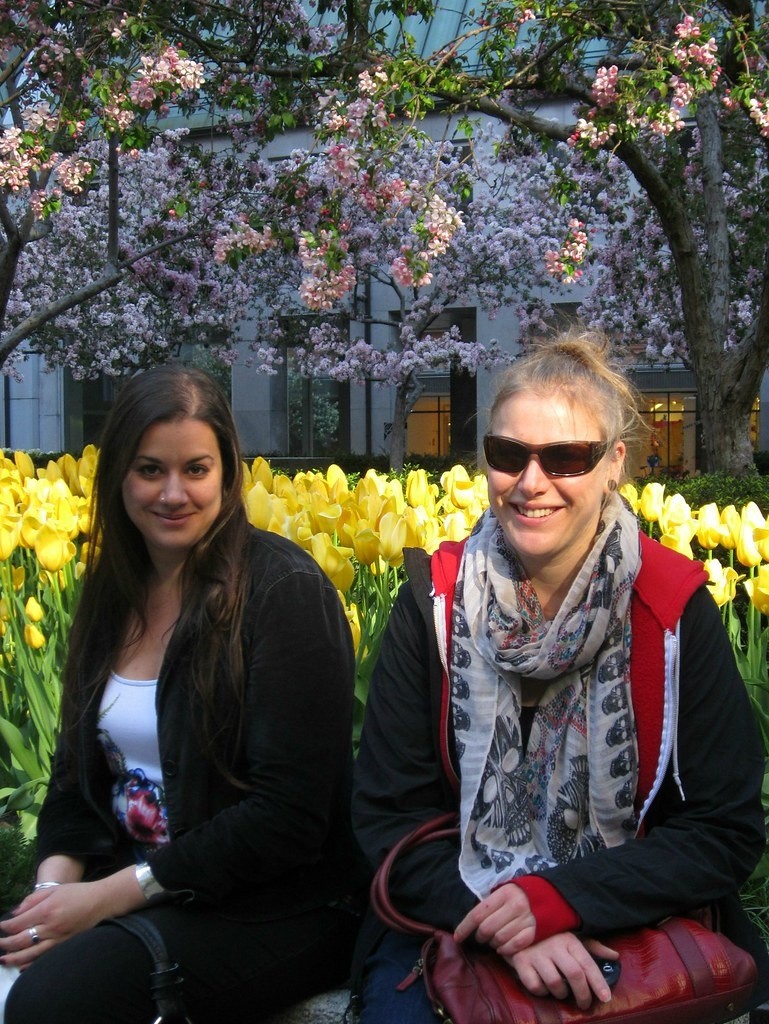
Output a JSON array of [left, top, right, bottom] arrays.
[[347, 330, 769, 1024], [0, 360, 357, 1024]]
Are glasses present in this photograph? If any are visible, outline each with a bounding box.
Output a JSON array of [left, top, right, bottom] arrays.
[[483, 430, 626, 478]]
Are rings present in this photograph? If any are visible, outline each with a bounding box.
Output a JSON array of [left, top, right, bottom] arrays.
[[28, 926, 40, 943]]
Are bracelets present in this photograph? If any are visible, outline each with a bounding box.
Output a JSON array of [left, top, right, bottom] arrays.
[[31, 881, 61, 889], [136, 859, 164, 900]]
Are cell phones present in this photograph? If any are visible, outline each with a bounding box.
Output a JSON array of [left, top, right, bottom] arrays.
[[511, 956, 621, 988]]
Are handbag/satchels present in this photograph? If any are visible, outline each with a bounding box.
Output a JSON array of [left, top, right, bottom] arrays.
[[368, 810, 765, 1024]]
